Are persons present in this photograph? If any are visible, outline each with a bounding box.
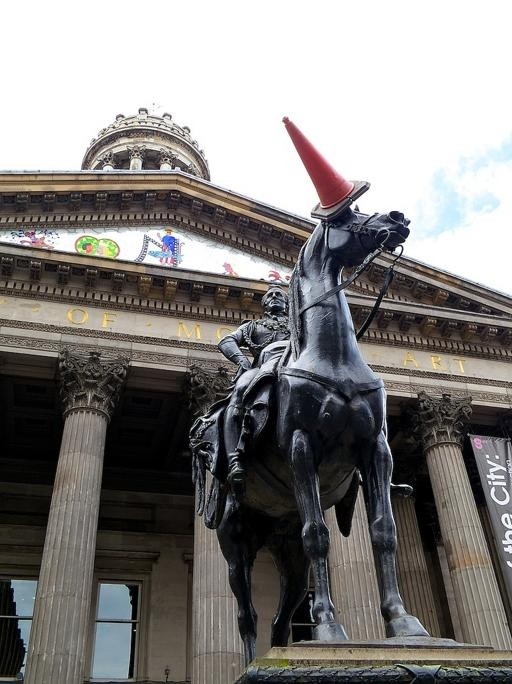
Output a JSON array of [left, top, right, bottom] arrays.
[[218, 285, 414, 497]]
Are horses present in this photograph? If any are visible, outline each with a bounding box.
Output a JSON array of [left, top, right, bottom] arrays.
[[217, 204, 431, 667]]
